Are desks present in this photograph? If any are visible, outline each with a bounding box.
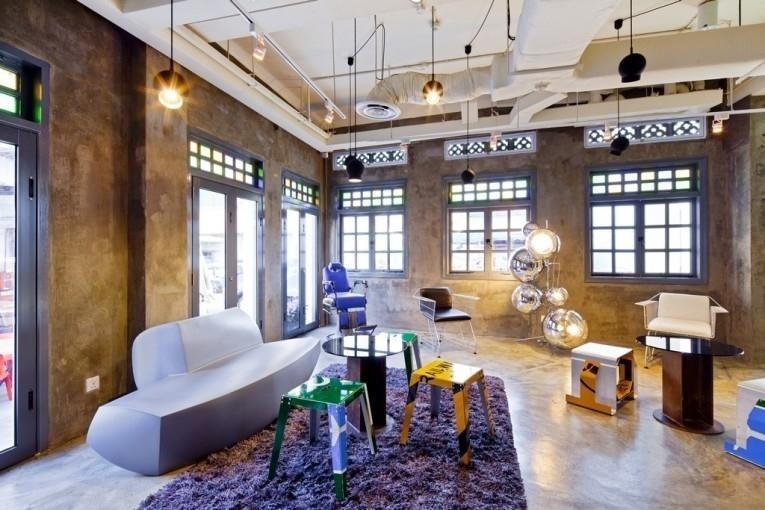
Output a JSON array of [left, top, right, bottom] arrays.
[[636, 335, 745, 436], [321, 336, 411, 430]]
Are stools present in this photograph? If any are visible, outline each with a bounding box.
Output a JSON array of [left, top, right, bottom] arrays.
[[723, 378, 765, 470], [566, 341, 640, 416], [397, 359, 497, 468], [266, 375, 378, 498], [377, 332, 422, 386]]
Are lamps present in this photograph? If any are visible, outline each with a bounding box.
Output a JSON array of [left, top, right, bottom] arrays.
[[420, 4, 446, 108], [457, 100, 478, 185], [149, 1, 195, 115], [607, 88, 631, 159], [340, 17, 368, 185], [616, 1, 648, 84]]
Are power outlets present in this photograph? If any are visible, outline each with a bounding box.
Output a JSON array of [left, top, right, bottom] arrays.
[[85, 375, 99, 393]]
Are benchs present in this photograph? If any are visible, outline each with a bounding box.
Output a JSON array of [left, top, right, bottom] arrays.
[[86, 307, 322, 477]]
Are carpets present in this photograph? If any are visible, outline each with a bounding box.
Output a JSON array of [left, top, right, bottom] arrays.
[[131, 364, 528, 509]]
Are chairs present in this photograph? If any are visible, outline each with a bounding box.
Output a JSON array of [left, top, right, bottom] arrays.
[[412, 286, 480, 359], [634, 292, 729, 369], [321, 262, 378, 341]]
